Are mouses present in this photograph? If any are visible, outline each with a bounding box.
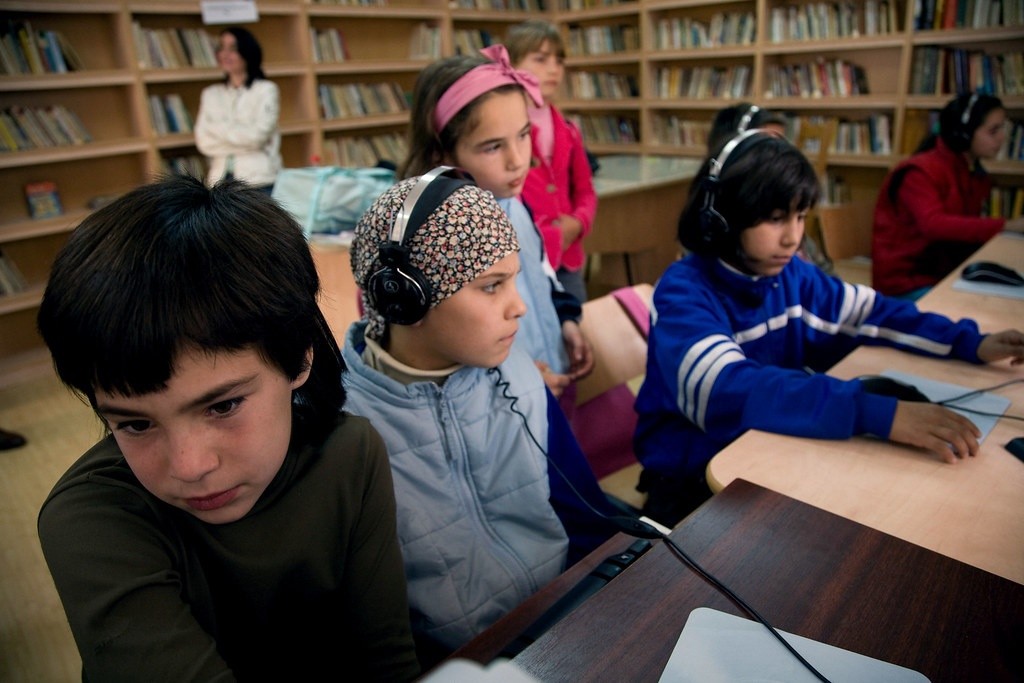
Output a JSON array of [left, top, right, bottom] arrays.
[[962, 261, 1024, 288], [851, 375, 930, 403]]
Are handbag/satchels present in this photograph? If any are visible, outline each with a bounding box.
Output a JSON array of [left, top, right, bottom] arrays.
[[271, 167, 402, 237]]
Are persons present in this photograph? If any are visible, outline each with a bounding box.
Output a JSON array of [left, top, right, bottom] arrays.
[[628, 129, 1023, 535], [195, 27, 281, 197], [706, 103, 826, 271], [336, 166, 569, 667], [38, 172, 422, 683], [872, 93, 1009, 302], [399, 20, 600, 400]]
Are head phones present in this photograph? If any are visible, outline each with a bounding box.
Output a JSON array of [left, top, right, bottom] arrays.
[[737, 105, 761, 133], [365, 165, 460, 326], [682, 130, 788, 257], [946, 94, 980, 154]]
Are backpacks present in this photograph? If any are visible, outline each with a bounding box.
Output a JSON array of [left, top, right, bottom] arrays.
[[553, 288, 653, 482]]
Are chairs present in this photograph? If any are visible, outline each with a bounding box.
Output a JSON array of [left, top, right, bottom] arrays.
[[818, 202, 873, 286], [573, 283, 656, 509]]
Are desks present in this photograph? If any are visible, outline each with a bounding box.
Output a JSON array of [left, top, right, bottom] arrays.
[[706, 231, 1024, 585], [305, 152, 704, 353], [441, 478, 1024, 683]]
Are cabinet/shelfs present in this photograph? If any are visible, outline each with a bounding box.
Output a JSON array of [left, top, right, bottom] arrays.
[[1, 0, 1023, 356]]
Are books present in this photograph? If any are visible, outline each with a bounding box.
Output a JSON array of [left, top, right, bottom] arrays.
[[135, 22, 219, 183], [448, 0, 643, 147], [651, 0, 1022, 269], [308, 1, 440, 170], [2, 17, 119, 297]]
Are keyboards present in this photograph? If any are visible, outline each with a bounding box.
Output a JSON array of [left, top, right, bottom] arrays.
[[496, 539, 653, 661]]
